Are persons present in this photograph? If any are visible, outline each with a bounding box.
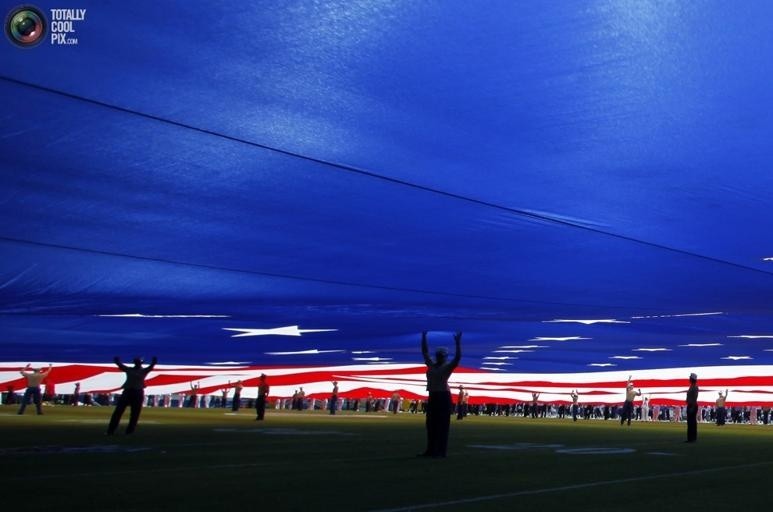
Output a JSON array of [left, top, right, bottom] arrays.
[[422, 329, 462, 459], [684, 373, 698, 443]]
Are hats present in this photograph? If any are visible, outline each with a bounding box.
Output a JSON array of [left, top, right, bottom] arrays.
[[434, 347, 450, 356], [690, 373, 698, 380]]
